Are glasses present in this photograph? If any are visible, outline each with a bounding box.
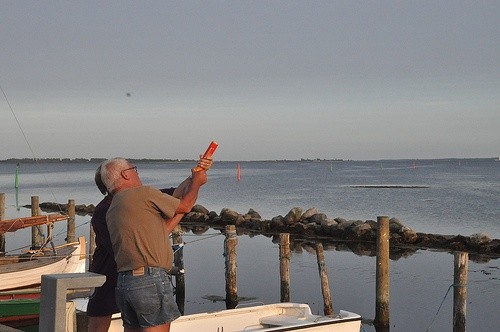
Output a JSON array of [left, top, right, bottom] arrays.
[[124, 166, 137, 173]]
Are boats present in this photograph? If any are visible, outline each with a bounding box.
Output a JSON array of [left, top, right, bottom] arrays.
[[0, 213, 96, 328], [143, 302, 363, 332]]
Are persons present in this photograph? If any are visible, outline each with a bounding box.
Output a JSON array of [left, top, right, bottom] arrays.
[[87, 154, 214, 332]]
